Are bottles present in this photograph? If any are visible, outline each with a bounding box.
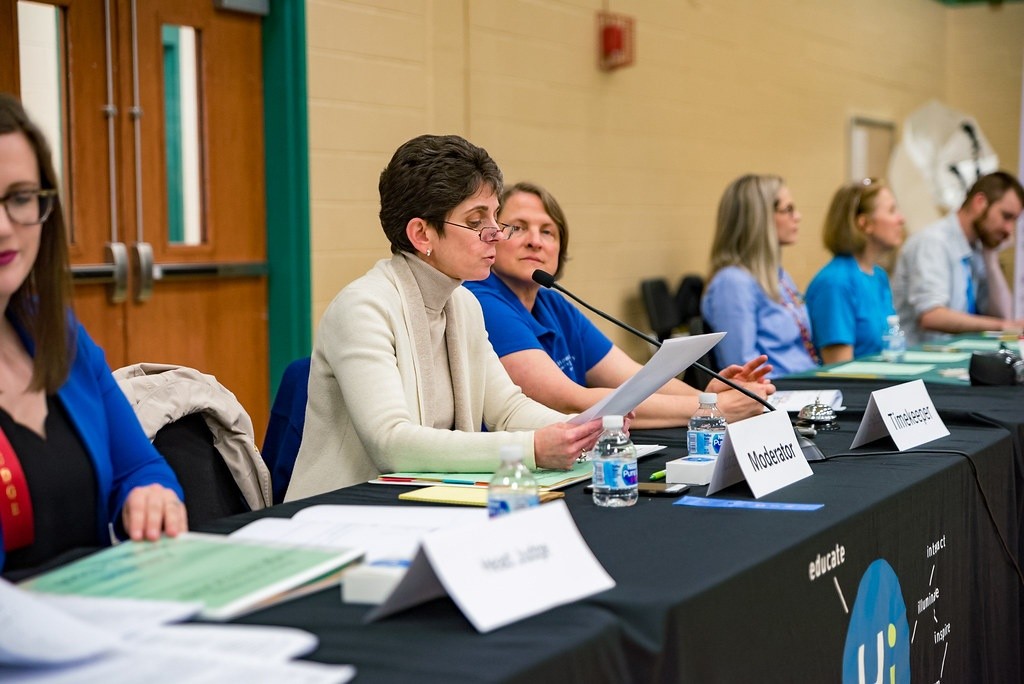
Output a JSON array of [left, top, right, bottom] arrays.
[[591, 414, 639, 508], [882, 314, 905, 364], [487, 446, 539, 520], [686, 393, 728, 456]]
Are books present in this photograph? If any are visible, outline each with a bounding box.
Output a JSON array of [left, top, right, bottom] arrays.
[[398, 487, 564, 506]]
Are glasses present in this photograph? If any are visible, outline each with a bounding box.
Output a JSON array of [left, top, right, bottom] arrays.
[[425, 217, 515, 242], [0, 187, 58, 227]]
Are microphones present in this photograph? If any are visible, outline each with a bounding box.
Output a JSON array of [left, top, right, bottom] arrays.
[[532, 268, 828, 461]]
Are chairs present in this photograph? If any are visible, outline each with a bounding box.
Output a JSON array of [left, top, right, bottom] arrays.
[[643, 275, 719, 389], [257, 352, 309, 501], [111, 363, 255, 524]]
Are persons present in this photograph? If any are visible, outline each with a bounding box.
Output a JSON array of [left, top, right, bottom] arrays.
[[283, 134, 636, 503], [890, 172, 1024, 344], [462, 183, 776, 428], [805, 178, 905, 365], [0, 94, 189, 566], [700, 175, 821, 378]]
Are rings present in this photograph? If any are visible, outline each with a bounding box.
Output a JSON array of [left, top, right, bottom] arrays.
[[579, 448, 586, 459]]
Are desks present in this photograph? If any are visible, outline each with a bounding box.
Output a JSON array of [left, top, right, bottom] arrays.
[[173, 378, 1024, 684]]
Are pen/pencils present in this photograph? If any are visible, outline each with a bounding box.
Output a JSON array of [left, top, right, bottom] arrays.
[[923, 345, 957, 352], [650, 470, 666, 480]]
